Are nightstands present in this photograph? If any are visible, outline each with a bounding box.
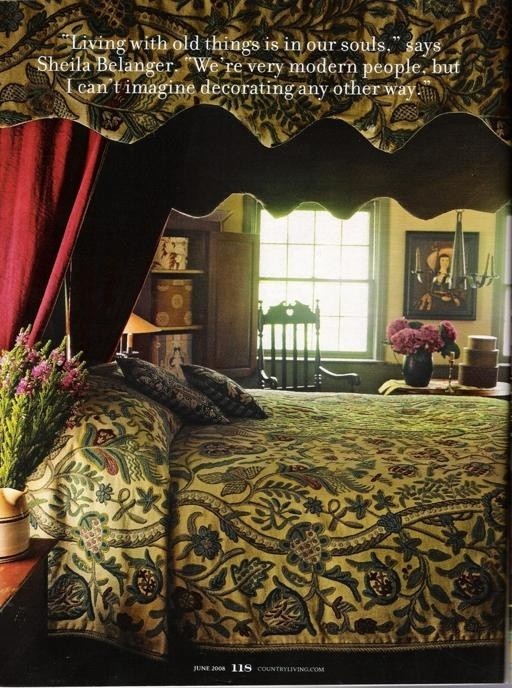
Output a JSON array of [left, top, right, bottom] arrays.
[[1, 537, 59, 688]]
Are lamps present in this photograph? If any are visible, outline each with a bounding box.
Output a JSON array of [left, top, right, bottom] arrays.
[[411, 209, 500, 299]]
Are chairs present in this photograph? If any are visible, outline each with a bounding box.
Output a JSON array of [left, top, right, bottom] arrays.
[[255, 297, 360, 392]]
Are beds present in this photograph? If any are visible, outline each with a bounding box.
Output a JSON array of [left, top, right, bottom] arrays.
[[28, 388, 510, 665]]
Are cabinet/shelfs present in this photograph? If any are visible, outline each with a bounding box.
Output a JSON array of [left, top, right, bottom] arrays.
[[132, 208, 260, 381]]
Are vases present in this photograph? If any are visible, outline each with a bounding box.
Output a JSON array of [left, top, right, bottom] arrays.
[[403, 350, 433, 387], [1, 485, 32, 565]]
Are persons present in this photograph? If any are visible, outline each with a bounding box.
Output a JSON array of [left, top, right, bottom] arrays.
[[415, 254, 457, 314], [169, 345, 184, 377]]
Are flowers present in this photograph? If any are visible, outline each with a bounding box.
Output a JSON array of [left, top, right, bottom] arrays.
[[381, 317, 460, 360], [1, 326, 90, 491]]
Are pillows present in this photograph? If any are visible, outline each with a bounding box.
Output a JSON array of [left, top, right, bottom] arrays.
[[117, 349, 271, 425]]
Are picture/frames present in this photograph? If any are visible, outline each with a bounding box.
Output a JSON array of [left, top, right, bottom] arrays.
[[402, 230, 479, 322]]
